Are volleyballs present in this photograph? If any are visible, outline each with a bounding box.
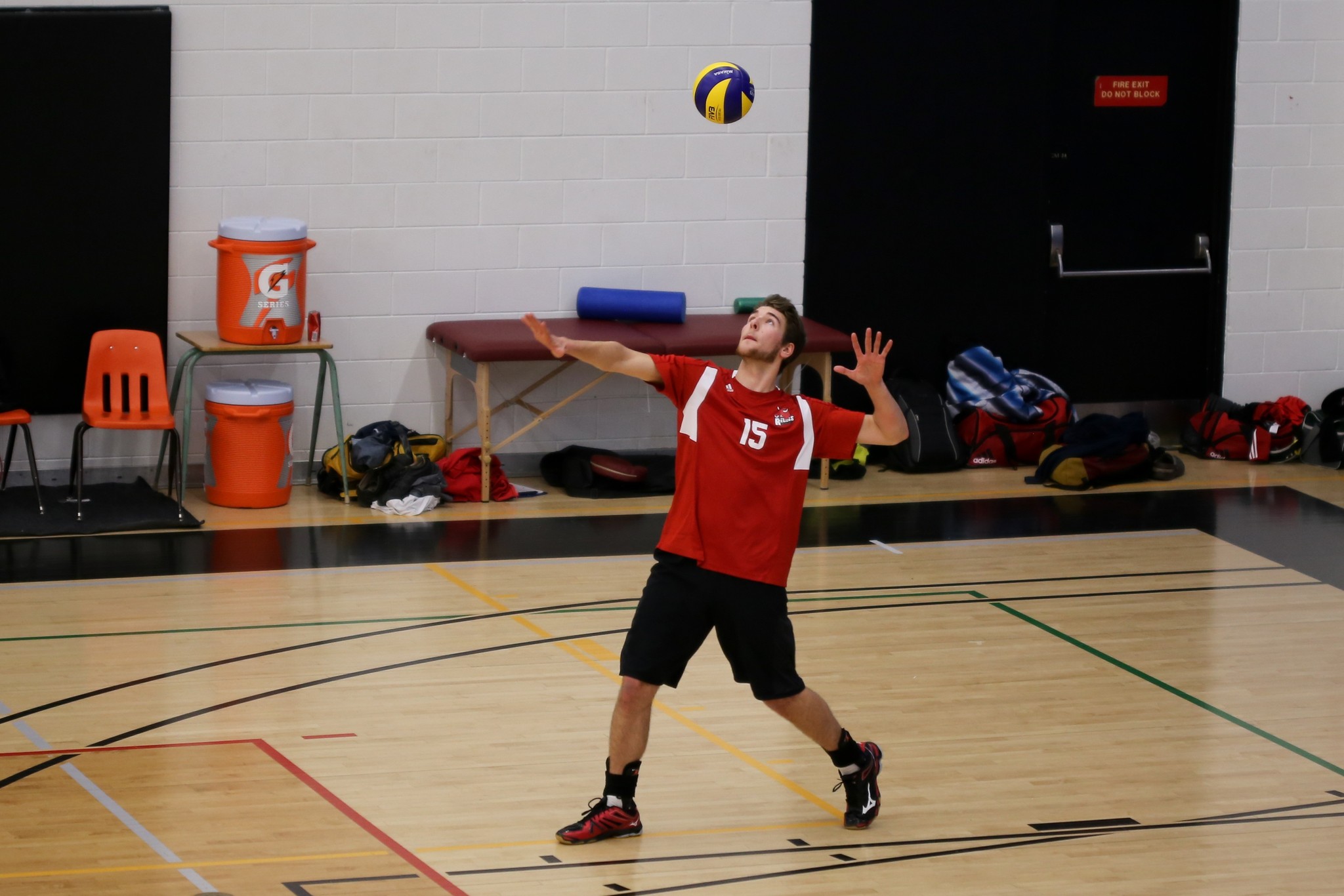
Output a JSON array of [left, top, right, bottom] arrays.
[[693, 62, 755, 124]]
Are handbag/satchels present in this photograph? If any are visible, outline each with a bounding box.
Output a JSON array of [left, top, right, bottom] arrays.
[[1300, 387, 1344, 469], [1039, 442, 1157, 489], [317, 434, 446, 501], [1180, 395, 1300, 464], [956, 398, 1075, 469]]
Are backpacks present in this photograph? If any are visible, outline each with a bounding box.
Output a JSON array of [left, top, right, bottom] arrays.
[[877, 368, 965, 475]]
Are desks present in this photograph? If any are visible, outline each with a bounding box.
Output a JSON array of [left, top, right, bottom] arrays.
[[150, 328, 351, 509], [423, 312, 856, 504]]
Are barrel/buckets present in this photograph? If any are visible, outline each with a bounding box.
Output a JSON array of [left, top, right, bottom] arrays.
[[208, 217, 316, 345], [205, 378, 295, 509]]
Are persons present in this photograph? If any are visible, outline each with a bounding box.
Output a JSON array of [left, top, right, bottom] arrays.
[[519, 293, 911, 848]]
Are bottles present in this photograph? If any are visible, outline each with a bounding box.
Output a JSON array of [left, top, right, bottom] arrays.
[[734, 297, 766, 314]]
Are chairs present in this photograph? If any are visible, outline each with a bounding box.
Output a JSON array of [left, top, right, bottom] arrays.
[[67, 327, 185, 525], [0, 408, 44, 520]]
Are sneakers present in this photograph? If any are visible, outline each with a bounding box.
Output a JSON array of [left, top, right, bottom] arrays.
[[833, 742, 884, 831], [555, 796, 643, 845]]
